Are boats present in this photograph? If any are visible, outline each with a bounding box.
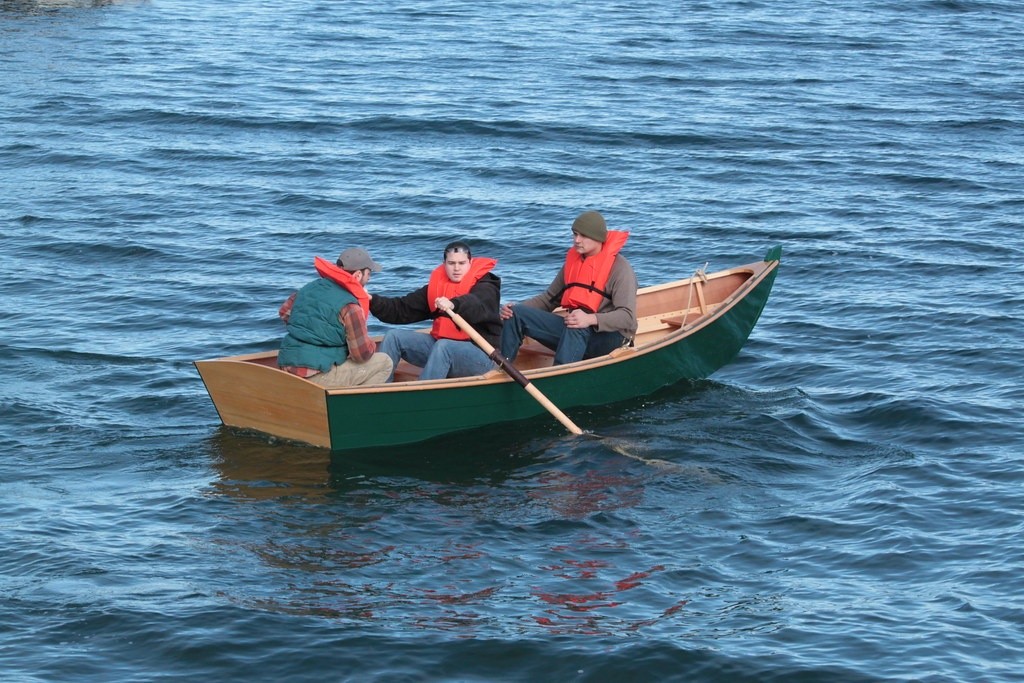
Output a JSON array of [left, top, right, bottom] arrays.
[[193, 243, 783, 452]]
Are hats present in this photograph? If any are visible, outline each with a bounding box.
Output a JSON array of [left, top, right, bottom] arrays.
[[335, 246, 383, 277], [572, 211, 606, 244]]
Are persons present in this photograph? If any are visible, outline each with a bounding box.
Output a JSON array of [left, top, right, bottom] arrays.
[[277, 247, 394, 389], [365, 241, 502, 381], [500, 209, 639, 368]]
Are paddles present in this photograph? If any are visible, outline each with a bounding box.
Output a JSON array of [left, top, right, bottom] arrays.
[[432, 300, 586, 436]]
